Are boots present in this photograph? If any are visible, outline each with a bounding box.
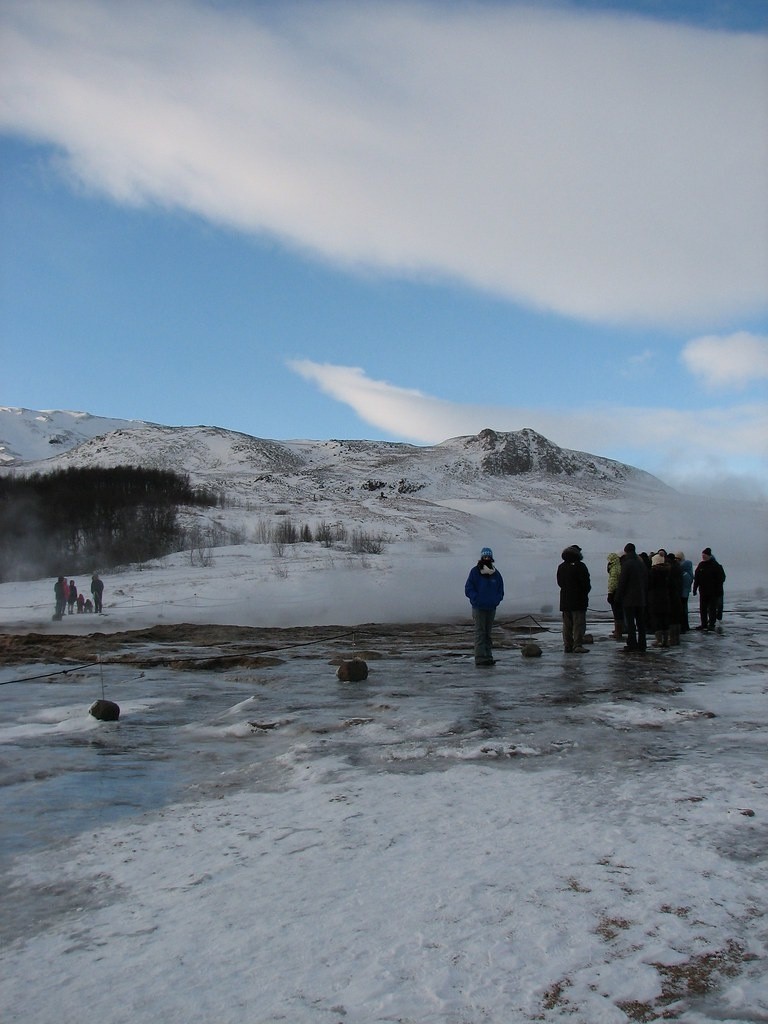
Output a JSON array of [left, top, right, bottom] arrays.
[[609, 621, 622, 638], [652, 631, 662, 646], [666, 630, 679, 646], [661, 632, 669, 647]]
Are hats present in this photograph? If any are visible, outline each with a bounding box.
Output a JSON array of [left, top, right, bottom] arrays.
[[624, 543, 635, 553], [674, 551, 684, 560], [652, 550, 664, 566], [481, 547, 492, 559], [702, 548, 712, 556], [572, 545, 582, 553]]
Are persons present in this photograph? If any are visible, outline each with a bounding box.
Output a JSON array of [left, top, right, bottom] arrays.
[[692, 548, 725, 630], [91, 575, 104, 613], [607, 543, 692, 652], [68, 580, 77, 614], [77, 594, 93, 613], [54, 576, 70, 616], [465, 548, 504, 667], [557, 545, 592, 653]]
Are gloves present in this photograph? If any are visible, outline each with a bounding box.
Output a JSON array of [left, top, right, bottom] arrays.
[[607, 593, 613, 604]]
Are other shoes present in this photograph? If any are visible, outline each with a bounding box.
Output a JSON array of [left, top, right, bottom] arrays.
[[619, 646, 637, 652], [638, 646, 647, 651], [564, 648, 572, 652], [573, 645, 590, 653], [476, 658, 495, 665], [696, 624, 707, 630], [708, 624, 716, 630]]
